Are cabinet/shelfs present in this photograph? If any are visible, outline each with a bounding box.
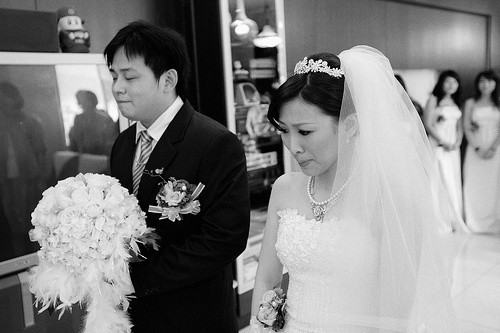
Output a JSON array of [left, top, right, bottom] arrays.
[[165, 0, 290, 219]]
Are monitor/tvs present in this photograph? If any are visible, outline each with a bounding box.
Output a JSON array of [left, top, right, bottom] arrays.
[[0, 52, 129, 278]]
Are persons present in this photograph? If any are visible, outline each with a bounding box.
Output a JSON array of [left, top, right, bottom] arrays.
[[251, 45, 500, 333], [463, 69, 499, 234], [422, 70, 463, 224], [102, 20, 251, 333], [68, 90, 119, 156]]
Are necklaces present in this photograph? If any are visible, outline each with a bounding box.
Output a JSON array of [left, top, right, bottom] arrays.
[[308, 166, 357, 221]]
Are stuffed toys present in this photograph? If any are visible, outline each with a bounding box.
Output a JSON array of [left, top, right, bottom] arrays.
[[56, 6, 91, 53]]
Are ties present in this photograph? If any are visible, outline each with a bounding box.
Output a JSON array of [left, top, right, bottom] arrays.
[[132, 130, 154, 197]]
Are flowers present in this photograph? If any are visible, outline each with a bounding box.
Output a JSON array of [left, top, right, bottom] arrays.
[[146, 169, 205, 222], [256, 288, 289, 333], [30, 174, 158, 333]]
